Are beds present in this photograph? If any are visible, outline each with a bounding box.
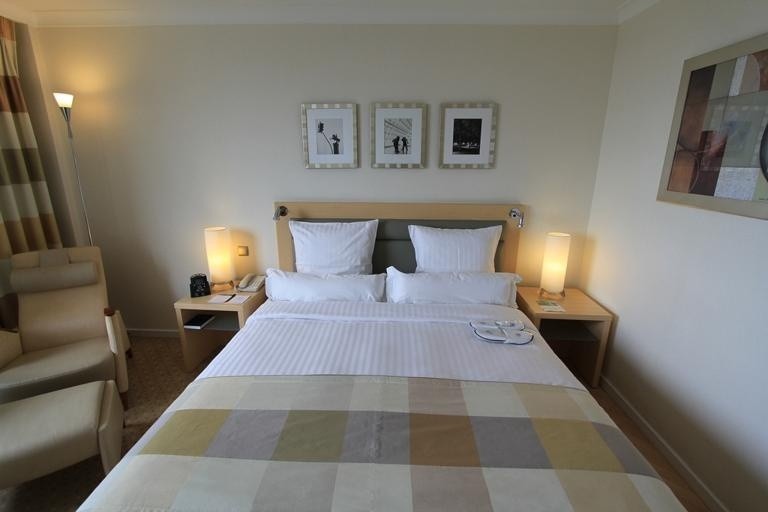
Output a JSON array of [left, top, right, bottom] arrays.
[[72, 199, 692, 512]]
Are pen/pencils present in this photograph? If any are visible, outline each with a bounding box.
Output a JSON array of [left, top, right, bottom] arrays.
[[224, 293, 236, 303]]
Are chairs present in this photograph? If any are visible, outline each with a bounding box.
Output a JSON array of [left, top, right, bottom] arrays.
[[1, 242, 132, 411]]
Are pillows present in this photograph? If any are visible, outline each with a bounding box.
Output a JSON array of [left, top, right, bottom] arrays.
[[288, 218, 380, 275], [384, 266, 524, 308], [406, 223, 504, 273], [264, 267, 386, 302]]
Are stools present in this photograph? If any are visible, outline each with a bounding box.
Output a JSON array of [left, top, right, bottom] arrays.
[[1, 377, 127, 493]]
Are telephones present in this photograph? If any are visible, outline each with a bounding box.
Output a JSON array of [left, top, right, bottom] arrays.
[[237, 273, 265, 292]]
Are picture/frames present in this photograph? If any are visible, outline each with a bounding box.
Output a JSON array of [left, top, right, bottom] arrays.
[[437, 101, 499, 170], [649, 29, 767, 222], [299, 102, 359, 171], [369, 100, 429, 169]]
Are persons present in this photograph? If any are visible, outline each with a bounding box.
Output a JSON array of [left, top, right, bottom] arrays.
[[401, 136, 408, 154], [391, 136, 401, 154]]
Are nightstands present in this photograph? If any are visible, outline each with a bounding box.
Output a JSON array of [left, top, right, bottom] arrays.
[[515, 285, 616, 388], [170, 272, 268, 374]]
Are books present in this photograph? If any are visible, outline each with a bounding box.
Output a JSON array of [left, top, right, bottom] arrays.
[[183, 313, 215, 330]]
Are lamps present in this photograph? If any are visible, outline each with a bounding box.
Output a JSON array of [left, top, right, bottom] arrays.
[[508, 207, 525, 229], [52, 91, 99, 247], [537, 232, 571, 297], [203, 226, 236, 290], [271, 204, 289, 226]]
